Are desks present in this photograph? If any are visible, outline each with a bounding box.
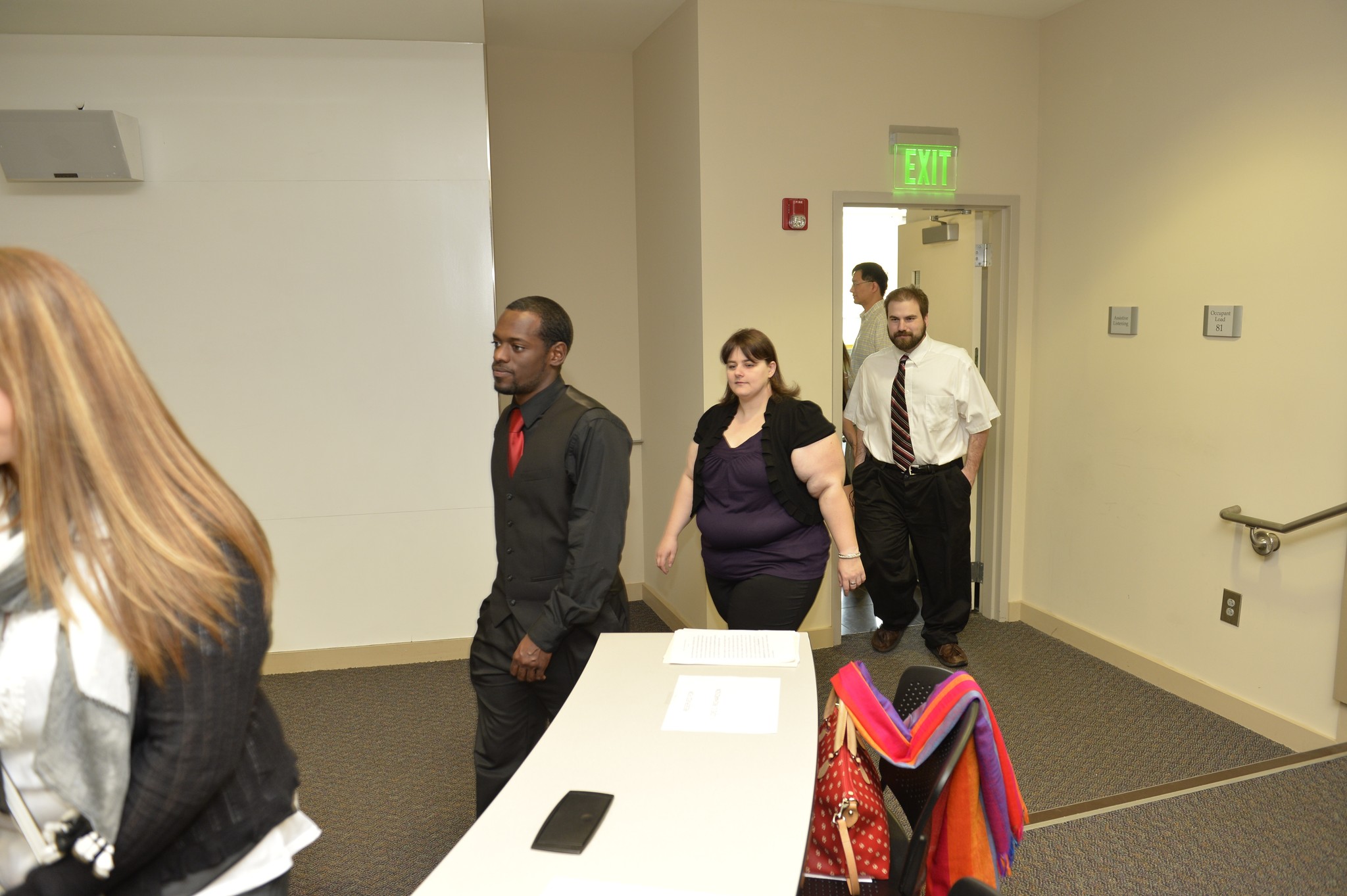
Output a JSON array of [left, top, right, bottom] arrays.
[[406, 631, 819, 896]]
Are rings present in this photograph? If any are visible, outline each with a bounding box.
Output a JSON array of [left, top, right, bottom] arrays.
[[850, 582, 856, 585]]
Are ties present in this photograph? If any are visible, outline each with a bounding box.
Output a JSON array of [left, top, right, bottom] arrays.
[[508, 408, 524, 479], [891, 355, 915, 471]]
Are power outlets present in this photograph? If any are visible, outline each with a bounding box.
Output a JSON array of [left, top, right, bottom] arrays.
[[1220, 588, 1243, 628]]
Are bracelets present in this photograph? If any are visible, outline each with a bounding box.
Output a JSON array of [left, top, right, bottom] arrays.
[[838, 552, 861, 559]]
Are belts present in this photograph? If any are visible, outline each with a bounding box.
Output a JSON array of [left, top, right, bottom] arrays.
[[866, 450, 956, 475]]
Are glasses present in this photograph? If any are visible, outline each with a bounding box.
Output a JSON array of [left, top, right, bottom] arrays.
[[852, 280, 874, 286]]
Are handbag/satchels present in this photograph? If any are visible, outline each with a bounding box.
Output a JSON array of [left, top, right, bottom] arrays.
[[804, 682, 890, 896]]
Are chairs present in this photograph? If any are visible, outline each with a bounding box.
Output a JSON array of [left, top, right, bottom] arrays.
[[799, 664, 1006, 896]]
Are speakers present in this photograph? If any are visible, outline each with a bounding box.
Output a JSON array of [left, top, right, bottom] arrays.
[[0, 108, 146, 182]]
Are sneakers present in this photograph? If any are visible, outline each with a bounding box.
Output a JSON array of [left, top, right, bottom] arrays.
[[924, 640, 969, 667], [871, 624, 906, 651]]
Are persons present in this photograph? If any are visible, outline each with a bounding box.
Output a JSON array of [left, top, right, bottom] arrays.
[[843, 343, 855, 524], [843, 262, 894, 600], [469, 295, 633, 820], [656, 327, 866, 632], [0, 246, 322, 896], [843, 284, 1002, 667]]
